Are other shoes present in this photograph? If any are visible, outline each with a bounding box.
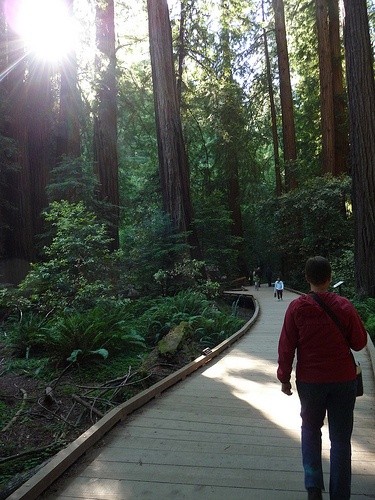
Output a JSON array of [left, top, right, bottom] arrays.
[[307, 488, 322, 500]]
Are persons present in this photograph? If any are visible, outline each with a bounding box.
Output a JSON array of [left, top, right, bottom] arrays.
[[274, 276, 284, 302], [277, 256, 368, 500], [254, 275, 260, 291]]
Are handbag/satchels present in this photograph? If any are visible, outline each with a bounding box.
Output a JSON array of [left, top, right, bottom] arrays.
[[354, 365, 363, 396], [273, 292, 276, 298]]
